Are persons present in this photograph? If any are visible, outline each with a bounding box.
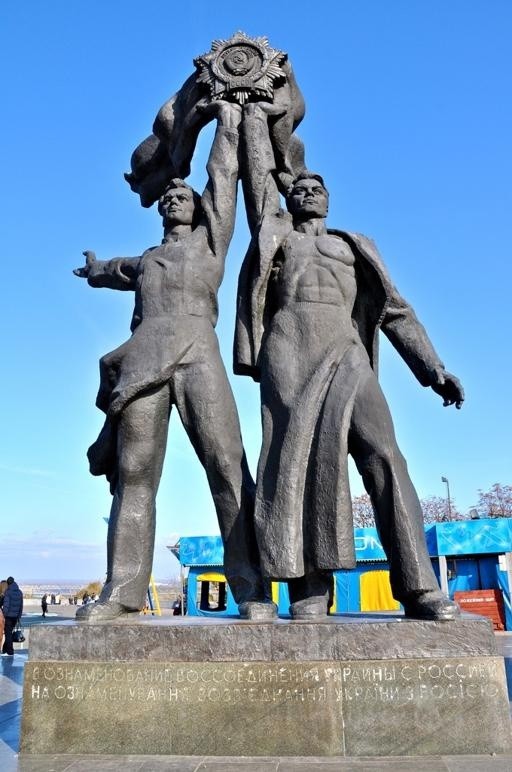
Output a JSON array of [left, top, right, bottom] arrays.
[[229, 100, 468, 621], [2, 576, 24, 655], [171, 595, 183, 615], [1, 579, 9, 651], [71, 100, 287, 623], [82, 589, 88, 605], [42, 592, 48, 617]]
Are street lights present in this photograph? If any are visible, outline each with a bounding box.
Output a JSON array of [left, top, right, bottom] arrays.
[[438, 476, 451, 521]]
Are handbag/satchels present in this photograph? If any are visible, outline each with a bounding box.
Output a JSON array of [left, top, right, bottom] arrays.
[[12, 630, 24, 643]]
